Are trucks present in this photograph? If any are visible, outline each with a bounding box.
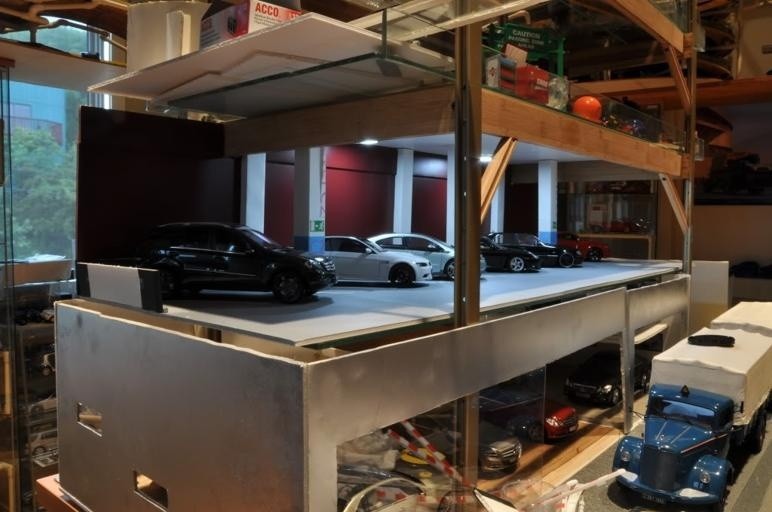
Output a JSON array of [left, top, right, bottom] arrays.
[[607, 300, 772, 511]]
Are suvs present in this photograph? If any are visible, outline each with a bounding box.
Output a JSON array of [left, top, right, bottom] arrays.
[[142, 217, 340, 305]]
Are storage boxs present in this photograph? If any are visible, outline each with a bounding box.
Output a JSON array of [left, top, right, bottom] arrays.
[[199, 0, 301, 50]]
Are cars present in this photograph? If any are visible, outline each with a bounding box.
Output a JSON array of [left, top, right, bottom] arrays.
[[367, 231, 490, 282], [13, 304, 60, 509], [325, 233, 434, 288], [472, 230, 612, 272], [336, 459, 458, 511], [416, 347, 657, 474]]
